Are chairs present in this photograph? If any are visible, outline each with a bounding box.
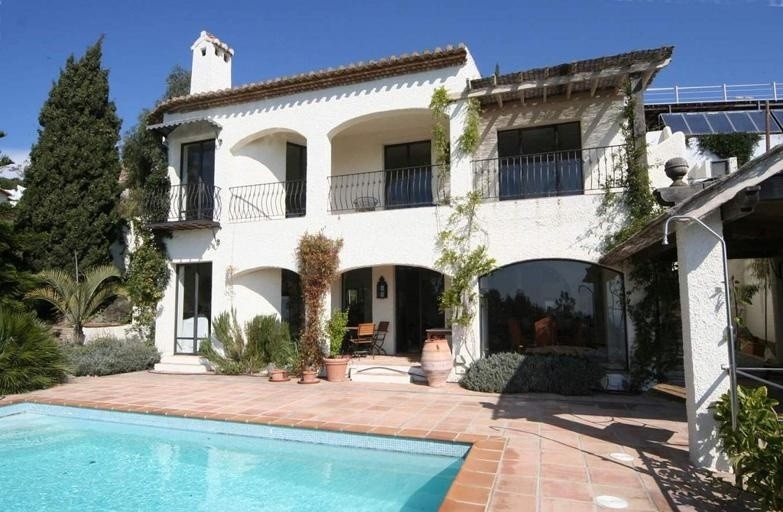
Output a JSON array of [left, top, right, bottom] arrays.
[[353, 196, 377, 212], [349, 320, 390, 356]]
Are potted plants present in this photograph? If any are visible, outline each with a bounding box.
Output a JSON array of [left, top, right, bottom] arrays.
[[419, 338, 453, 387], [267, 305, 351, 383]]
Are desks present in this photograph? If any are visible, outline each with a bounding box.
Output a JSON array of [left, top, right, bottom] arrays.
[[424, 327, 451, 339]]
